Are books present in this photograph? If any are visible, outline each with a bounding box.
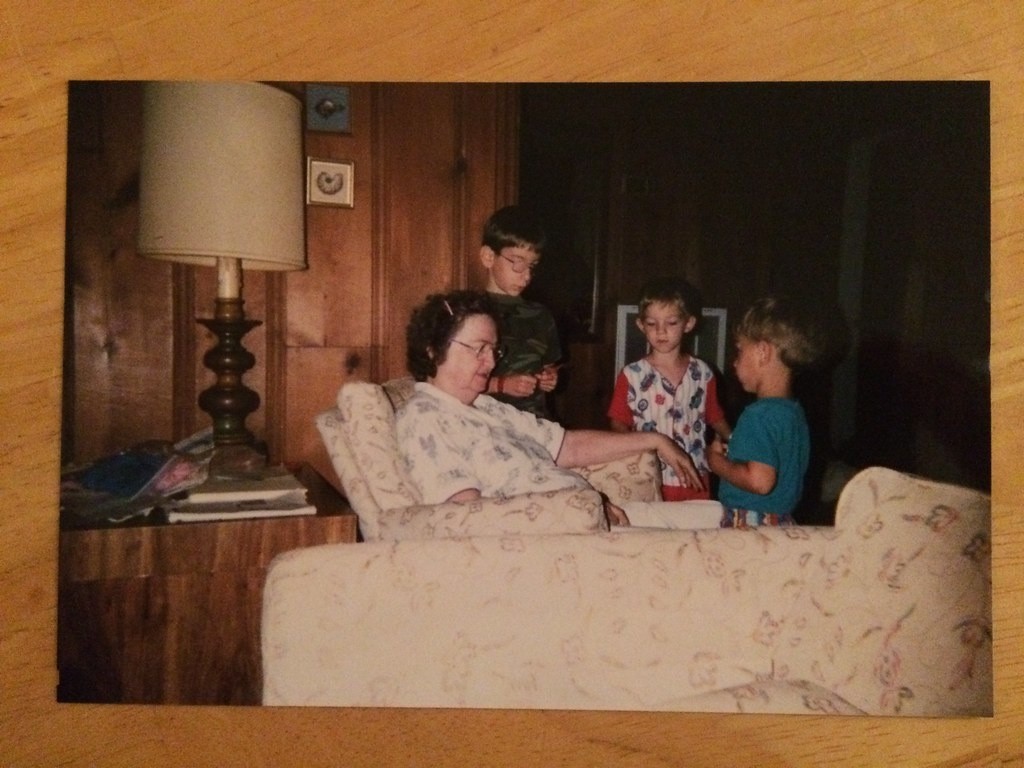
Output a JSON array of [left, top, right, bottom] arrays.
[[163, 472, 317, 524]]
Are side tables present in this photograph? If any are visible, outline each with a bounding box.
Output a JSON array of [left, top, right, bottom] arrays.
[[59, 462, 358, 708]]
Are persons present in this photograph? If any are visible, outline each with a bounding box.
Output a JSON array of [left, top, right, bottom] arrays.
[[603, 280, 731, 500], [390, 289, 704, 528], [707, 296, 828, 531], [477, 206, 569, 423]]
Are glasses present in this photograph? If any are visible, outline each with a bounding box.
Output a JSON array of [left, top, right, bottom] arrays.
[[449, 340, 506, 363], [493, 250, 538, 274]]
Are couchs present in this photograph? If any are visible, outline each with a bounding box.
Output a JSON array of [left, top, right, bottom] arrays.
[[257, 464, 993, 719], [315, 375, 663, 542]]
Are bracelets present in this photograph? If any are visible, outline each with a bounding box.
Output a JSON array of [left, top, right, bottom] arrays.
[[497, 376, 505, 393]]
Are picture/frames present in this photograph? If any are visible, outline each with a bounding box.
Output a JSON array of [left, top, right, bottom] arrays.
[[614, 305, 727, 386], [520, 118, 614, 344]]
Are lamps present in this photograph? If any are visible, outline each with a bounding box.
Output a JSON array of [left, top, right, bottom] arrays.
[[135, 81, 307, 470]]
